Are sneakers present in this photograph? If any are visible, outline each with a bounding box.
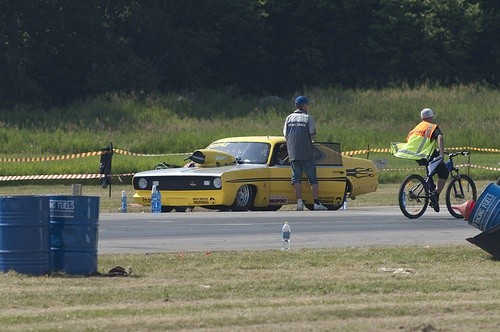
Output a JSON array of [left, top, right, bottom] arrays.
[[314, 202, 328, 210], [297, 204, 303, 211]]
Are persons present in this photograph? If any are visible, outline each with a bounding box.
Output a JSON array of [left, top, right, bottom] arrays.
[[282, 96, 329, 211], [390, 107, 449, 212]]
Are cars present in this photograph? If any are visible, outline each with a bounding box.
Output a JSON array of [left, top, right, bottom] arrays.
[[131, 133, 380, 214]]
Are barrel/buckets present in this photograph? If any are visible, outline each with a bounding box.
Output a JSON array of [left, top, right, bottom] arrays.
[[48, 193, 101, 275], [467, 181, 500, 231], [0, 193, 50, 276]]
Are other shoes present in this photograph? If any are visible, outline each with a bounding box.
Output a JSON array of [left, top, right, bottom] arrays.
[[429, 191, 439, 212], [430, 184, 436, 192]]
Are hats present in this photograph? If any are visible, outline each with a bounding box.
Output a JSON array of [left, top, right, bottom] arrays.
[[421, 108, 434, 118], [295, 96, 311, 105]]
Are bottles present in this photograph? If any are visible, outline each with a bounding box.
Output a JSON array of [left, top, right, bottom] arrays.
[[121, 190, 127, 213], [151, 184, 158, 213], [156, 189, 162, 213], [281, 221, 291, 251]]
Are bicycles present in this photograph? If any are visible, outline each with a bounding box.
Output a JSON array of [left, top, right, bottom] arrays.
[[396, 149, 479, 220]]
